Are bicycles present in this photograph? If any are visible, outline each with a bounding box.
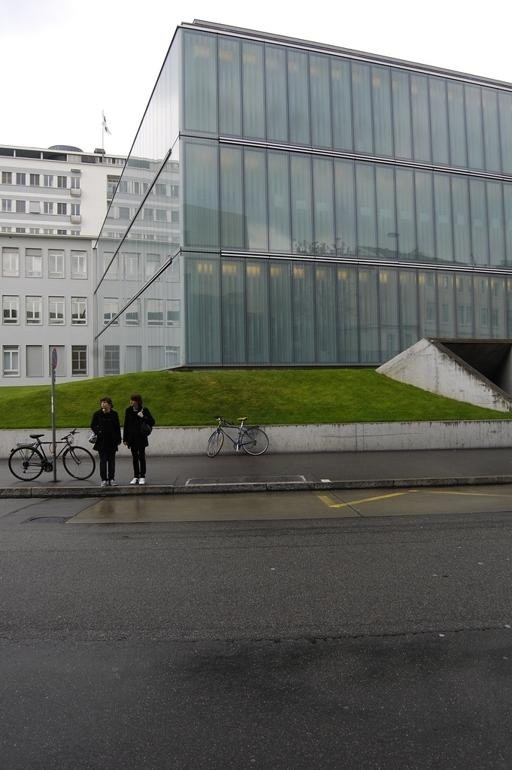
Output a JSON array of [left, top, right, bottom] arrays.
[[206, 416, 269, 459], [8, 428, 95, 481]]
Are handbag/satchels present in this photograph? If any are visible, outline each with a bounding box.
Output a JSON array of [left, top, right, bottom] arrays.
[[88, 432, 98, 444], [139, 421, 153, 436]]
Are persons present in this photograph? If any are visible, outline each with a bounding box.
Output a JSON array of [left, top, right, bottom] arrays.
[[88, 395, 120, 487], [122, 394, 156, 485]]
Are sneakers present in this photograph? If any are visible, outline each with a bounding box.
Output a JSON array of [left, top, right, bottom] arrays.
[[129, 478, 146, 485], [100, 479, 119, 487]]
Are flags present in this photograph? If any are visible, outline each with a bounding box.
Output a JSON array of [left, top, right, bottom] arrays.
[[101, 110, 114, 136]]
